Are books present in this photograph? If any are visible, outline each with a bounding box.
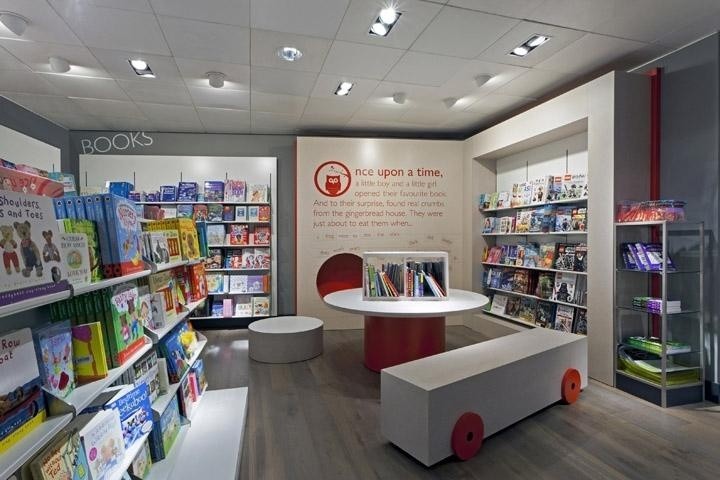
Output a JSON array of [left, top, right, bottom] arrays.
[[630, 294, 682, 313], [477, 171, 589, 335], [364, 258, 448, 300], [615, 196, 687, 229], [617, 239, 679, 273], [210, 180, 272, 318], [0, 157, 211, 479]]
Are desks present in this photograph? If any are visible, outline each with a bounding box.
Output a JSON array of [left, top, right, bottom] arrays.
[[323, 288, 489, 374]]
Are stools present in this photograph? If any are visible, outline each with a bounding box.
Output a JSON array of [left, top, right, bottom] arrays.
[[248, 316, 323, 364]]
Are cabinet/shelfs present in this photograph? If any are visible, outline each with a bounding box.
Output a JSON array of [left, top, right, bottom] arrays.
[[134, 193, 271, 320], [1, 219, 249, 478], [613, 220, 706, 408], [361, 250, 450, 301], [481, 196, 588, 328]]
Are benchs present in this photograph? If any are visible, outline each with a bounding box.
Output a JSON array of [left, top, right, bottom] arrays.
[[380, 327, 588, 467]]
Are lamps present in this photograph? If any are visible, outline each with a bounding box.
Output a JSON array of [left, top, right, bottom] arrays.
[[393, 74, 492, 109], [207, 72, 224, 88], [0, 12, 71, 73]]
